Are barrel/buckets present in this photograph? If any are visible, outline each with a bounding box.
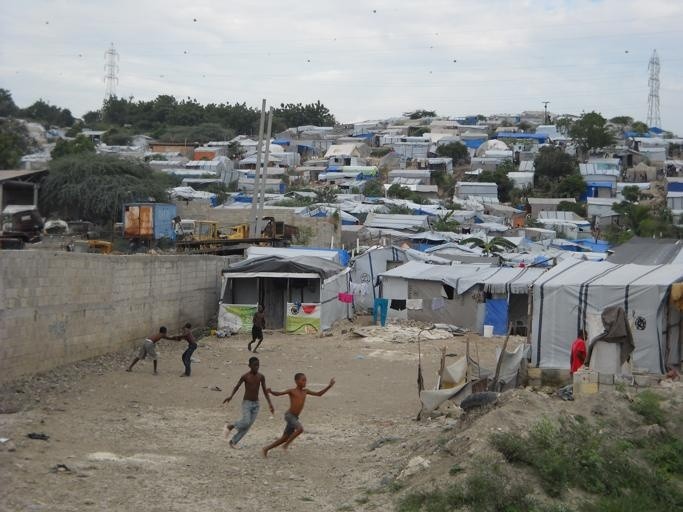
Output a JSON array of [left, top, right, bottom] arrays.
[[527, 368, 543, 388]]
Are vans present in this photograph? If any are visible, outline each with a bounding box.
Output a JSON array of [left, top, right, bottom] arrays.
[[173, 218, 198, 240]]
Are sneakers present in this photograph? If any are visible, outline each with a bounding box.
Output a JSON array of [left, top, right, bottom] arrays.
[[232, 443, 241, 449], [224, 423, 231, 439]]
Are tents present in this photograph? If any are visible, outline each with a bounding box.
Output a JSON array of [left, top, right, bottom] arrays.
[[20, 108, 682, 385]]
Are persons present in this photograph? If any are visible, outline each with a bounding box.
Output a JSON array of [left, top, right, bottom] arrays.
[[262, 372, 335, 457], [222, 357, 274, 449]]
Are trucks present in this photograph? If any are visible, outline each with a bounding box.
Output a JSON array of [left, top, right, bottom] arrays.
[[121, 197, 180, 251]]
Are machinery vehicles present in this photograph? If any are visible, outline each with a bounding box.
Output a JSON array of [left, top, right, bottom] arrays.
[[189, 220, 250, 244]]
